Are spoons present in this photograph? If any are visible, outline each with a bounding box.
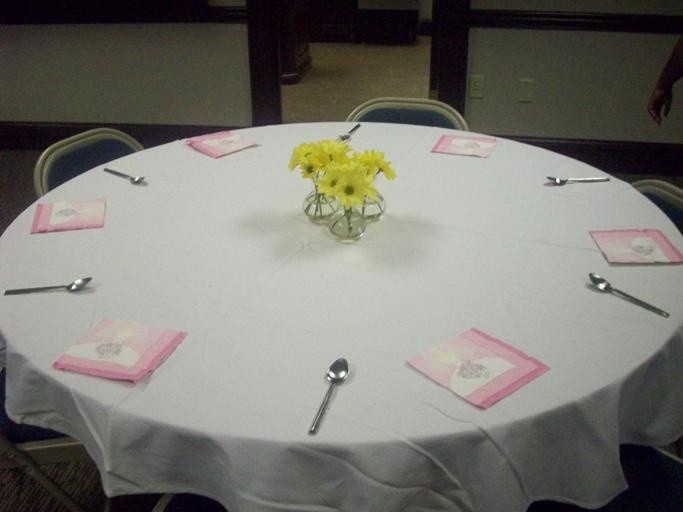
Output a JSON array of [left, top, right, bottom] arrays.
[[105, 167, 146, 185], [7, 277, 91, 294], [590, 274, 670, 318], [307, 358, 350, 434], [548, 176, 609, 187], [341, 123, 360, 140]]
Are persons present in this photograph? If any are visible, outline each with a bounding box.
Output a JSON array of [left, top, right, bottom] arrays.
[[648, 32, 683, 125]]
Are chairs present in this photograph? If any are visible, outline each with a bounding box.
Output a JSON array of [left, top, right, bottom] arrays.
[[33, 127, 143, 199], [631, 178, 683, 235], [344, 96, 471, 133]]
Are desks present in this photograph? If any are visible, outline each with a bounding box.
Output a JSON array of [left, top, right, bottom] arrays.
[[2, 120, 682, 511]]
